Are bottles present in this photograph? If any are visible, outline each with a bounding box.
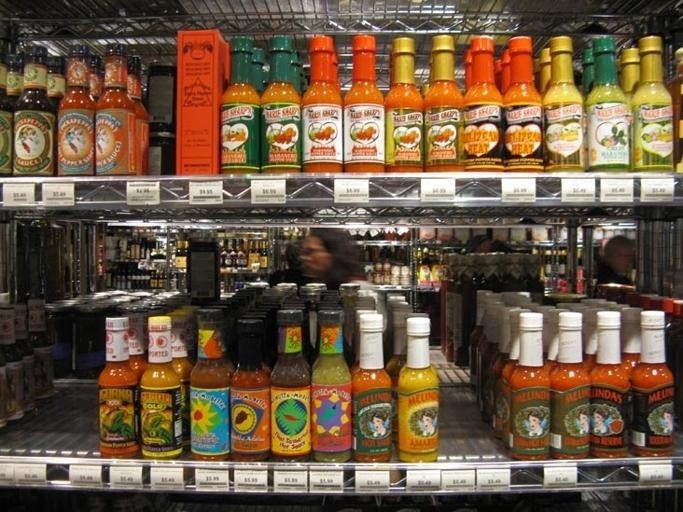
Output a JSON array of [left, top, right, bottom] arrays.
[[1, 226, 683, 463], [0, 36, 176, 178], [219, 32, 683, 176]]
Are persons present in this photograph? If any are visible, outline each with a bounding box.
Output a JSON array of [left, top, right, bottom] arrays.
[[269, 245, 310, 286], [299, 228, 366, 288], [593, 235, 634, 298]]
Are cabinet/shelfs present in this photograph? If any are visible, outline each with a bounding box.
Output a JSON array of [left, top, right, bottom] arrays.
[[0, 0, 683, 510]]
